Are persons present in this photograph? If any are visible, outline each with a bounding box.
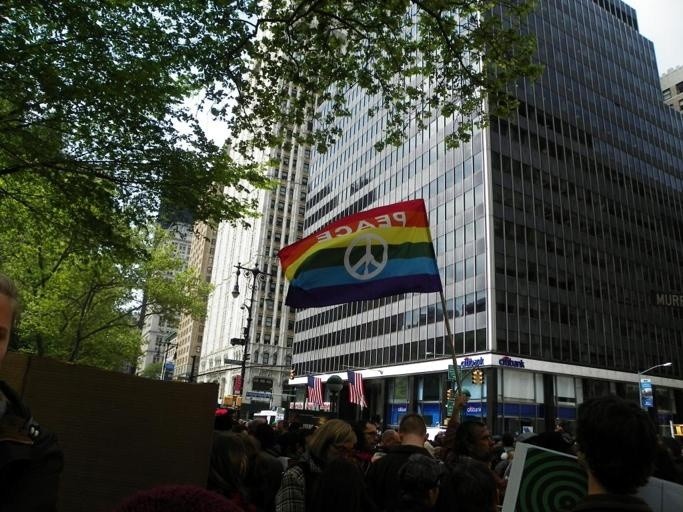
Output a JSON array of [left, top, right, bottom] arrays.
[[0, 273, 65, 512], [116, 385, 683, 512]]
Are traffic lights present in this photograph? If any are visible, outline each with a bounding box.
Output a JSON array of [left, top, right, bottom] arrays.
[[236, 397, 243, 406], [230, 338, 245, 345], [471, 371, 485, 384], [289, 370, 296, 379], [446, 389, 453, 399]]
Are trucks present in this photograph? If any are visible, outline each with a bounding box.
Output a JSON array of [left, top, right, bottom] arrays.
[[253, 410, 284, 424]]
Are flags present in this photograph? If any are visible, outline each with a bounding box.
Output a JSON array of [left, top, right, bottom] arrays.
[[305, 373, 324, 406], [346, 369, 368, 407], [274, 197, 443, 308]]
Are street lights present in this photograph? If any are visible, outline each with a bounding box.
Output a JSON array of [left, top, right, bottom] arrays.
[[326, 375, 344, 412], [426, 352, 455, 399], [232, 262, 274, 418], [160, 341, 179, 380], [638, 362, 672, 409]]
